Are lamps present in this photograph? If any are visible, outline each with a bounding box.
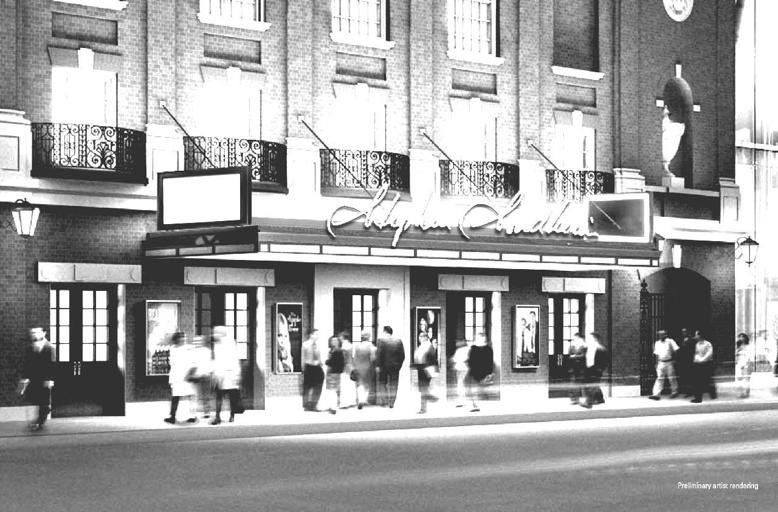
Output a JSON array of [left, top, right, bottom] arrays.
[[730, 235, 760, 268], [0, 198, 41, 240]]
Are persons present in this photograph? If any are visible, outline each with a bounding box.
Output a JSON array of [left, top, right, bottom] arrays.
[[416, 307, 439, 364], [581, 331, 607, 408], [567, 332, 587, 404], [17, 325, 57, 429], [647, 329, 681, 398], [735, 332, 751, 398], [676, 328, 696, 398], [275, 313, 294, 372], [519, 310, 539, 367], [299, 326, 494, 414], [164, 326, 242, 424], [688, 329, 716, 402]]
